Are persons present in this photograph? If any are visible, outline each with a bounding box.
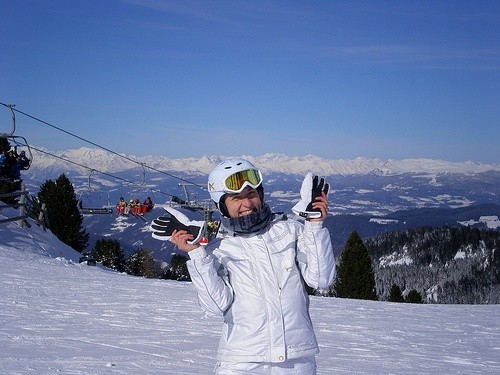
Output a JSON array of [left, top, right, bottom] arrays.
[[170, 158, 335, 375], [0, 147, 29, 181], [205, 218, 219, 234], [116, 197, 153, 215]]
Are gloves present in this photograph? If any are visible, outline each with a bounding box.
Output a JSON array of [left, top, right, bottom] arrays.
[[291, 172, 331, 219], [150, 206, 207, 245]]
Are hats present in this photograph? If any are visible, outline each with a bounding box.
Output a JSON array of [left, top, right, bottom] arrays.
[[130, 199, 134, 204]]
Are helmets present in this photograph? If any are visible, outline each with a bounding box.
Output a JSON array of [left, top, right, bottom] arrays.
[[208, 159, 265, 219]]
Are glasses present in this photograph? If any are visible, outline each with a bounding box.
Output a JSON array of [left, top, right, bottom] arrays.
[[208, 167, 263, 194]]
